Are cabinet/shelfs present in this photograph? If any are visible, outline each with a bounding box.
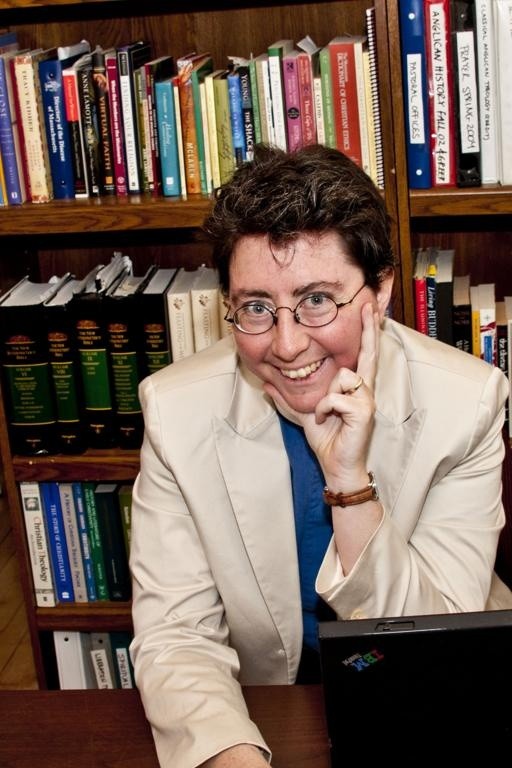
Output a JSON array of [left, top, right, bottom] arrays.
[[0, 0, 512, 689]]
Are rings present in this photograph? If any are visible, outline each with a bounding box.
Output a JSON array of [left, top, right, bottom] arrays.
[[348, 378, 362, 395]]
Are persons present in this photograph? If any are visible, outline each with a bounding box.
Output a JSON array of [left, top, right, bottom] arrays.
[[132, 152, 512, 768]]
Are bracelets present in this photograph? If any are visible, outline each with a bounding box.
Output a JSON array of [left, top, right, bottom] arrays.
[[320, 473, 376, 507]]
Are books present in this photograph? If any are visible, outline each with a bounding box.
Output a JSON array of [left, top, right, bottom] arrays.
[[1, 1, 512, 206], [20, 480, 135, 690], [1, 253, 233, 456], [417, 245, 512, 438]]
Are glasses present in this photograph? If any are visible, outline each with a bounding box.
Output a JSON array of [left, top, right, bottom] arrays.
[[224, 284, 367, 335]]
